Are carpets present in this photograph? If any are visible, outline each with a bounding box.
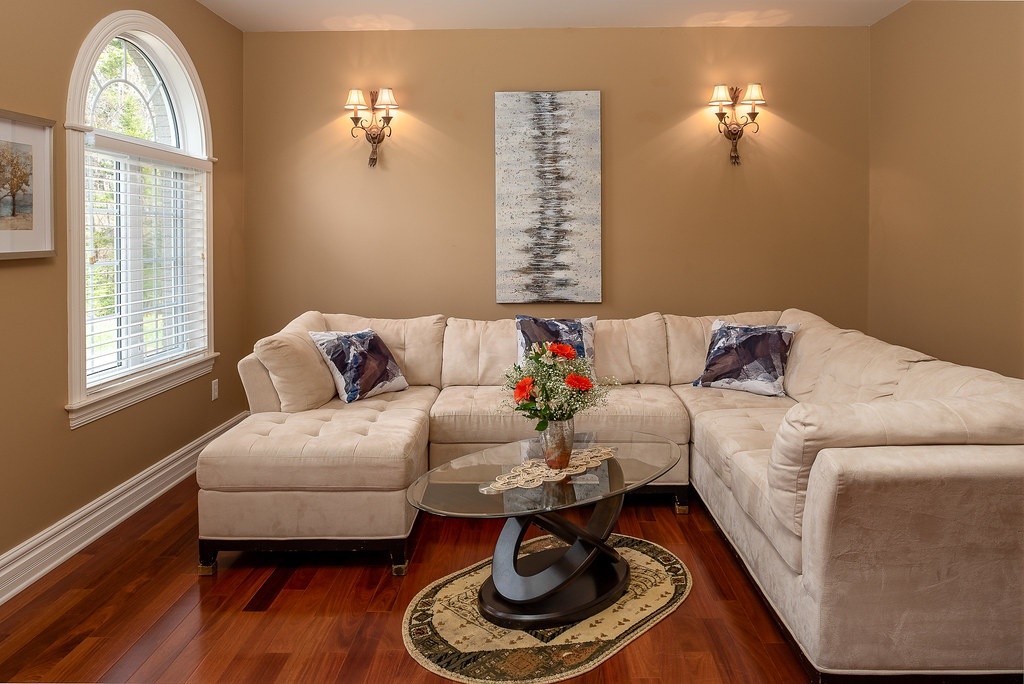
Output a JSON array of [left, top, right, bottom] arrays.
[[402, 532, 693, 684]]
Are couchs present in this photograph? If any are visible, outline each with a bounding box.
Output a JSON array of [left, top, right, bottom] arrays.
[[196, 309, 1023, 684]]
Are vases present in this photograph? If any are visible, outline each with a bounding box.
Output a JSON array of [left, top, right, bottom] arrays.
[[537, 418, 574, 469]]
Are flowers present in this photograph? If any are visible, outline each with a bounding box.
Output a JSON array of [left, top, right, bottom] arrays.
[[497, 340, 623, 432]]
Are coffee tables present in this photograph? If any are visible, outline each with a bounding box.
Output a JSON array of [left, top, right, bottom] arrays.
[[405, 428, 682, 630]]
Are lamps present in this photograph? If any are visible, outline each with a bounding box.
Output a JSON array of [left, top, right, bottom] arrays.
[[707, 82, 766, 166], [345, 88, 398, 167]]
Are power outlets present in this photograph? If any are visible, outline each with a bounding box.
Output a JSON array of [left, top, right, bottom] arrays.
[[211, 379, 218, 401]]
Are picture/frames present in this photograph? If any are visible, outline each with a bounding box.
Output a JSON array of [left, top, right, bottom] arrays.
[[0, 109, 58, 261]]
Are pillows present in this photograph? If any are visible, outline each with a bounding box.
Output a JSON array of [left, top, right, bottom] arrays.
[[692, 319, 796, 398], [514, 313, 600, 392], [308, 327, 410, 404]]
